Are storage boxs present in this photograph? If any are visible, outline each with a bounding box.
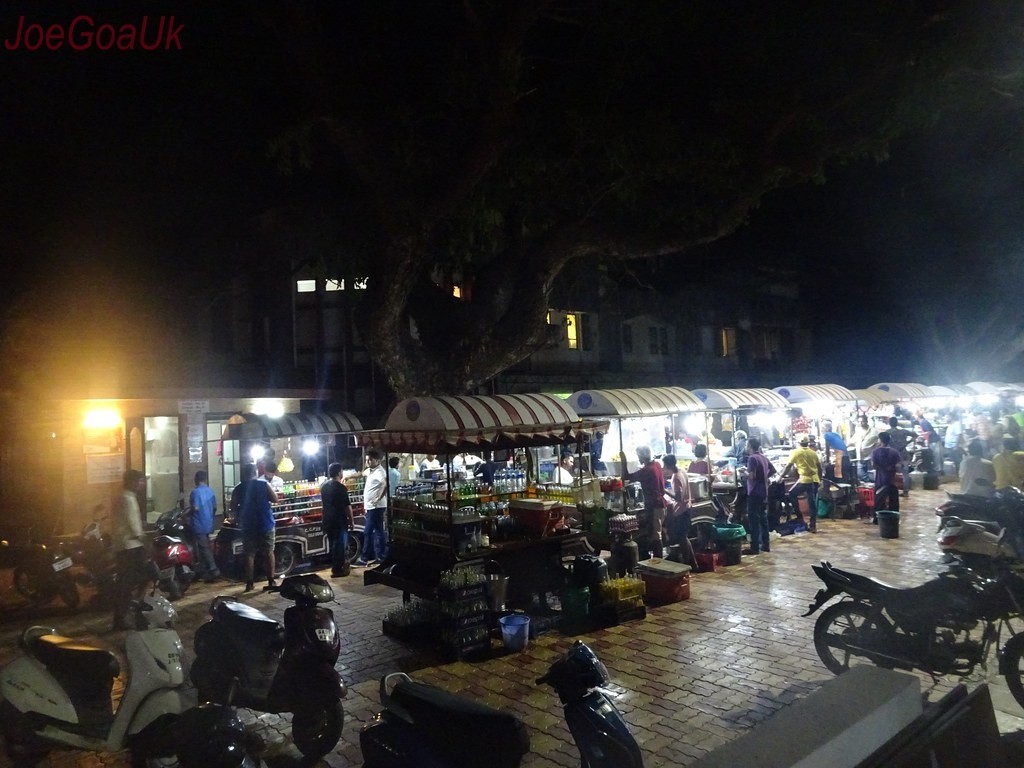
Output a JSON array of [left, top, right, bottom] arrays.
[[858, 488, 873, 506], [639, 560, 690, 602], [696, 551, 727, 571], [509, 501, 562, 535]]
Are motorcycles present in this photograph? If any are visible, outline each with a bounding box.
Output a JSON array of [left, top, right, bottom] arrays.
[[150, 492, 206, 601], [799, 526, 1024, 709], [933, 477, 1024, 571]]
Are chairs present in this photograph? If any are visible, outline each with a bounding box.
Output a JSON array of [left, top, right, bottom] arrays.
[[818, 479, 854, 518]]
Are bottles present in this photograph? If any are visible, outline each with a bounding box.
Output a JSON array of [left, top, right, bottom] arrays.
[[382, 464, 646, 643], [264, 468, 369, 530]]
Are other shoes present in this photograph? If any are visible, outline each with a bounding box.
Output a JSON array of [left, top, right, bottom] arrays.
[[761, 545, 770, 552], [112, 620, 136, 630], [245, 584, 255, 592], [865, 519, 878, 525], [742, 548, 759, 556], [269, 582, 276, 590], [353, 560, 367, 566], [369, 559, 384, 565], [899, 494, 908, 498], [809, 527, 816, 533]]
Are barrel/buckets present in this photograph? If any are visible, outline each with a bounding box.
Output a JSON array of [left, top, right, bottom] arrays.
[[796, 496, 829, 518], [498, 614, 530, 651], [875, 510, 900, 539]]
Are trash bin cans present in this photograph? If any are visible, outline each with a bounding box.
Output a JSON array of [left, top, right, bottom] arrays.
[[875, 509, 899, 538], [556, 555, 607, 619], [713, 523, 747, 567]]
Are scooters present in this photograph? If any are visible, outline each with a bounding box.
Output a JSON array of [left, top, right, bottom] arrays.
[[53, 504, 127, 610], [357, 639, 645, 768], [0, 559, 267, 768], [186, 575, 350, 758], [0, 511, 81, 607]]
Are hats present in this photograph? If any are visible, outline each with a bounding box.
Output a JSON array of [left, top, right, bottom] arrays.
[[800, 435, 810, 444]]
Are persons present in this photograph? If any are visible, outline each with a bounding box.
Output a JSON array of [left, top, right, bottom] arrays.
[[620, 429, 777, 563], [321, 463, 354, 573], [421, 454, 440, 476], [229, 463, 279, 591], [453, 453, 484, 471], [344, 450, 389, 568], [187, 470, 221, 581], [780, 404, 1024, 534], [257, 462, 283, 487], [389, 457, 401, 497], [110, 470, 154, 631], [552, 451, 574, 484], [476, 453, 499, 484]]
